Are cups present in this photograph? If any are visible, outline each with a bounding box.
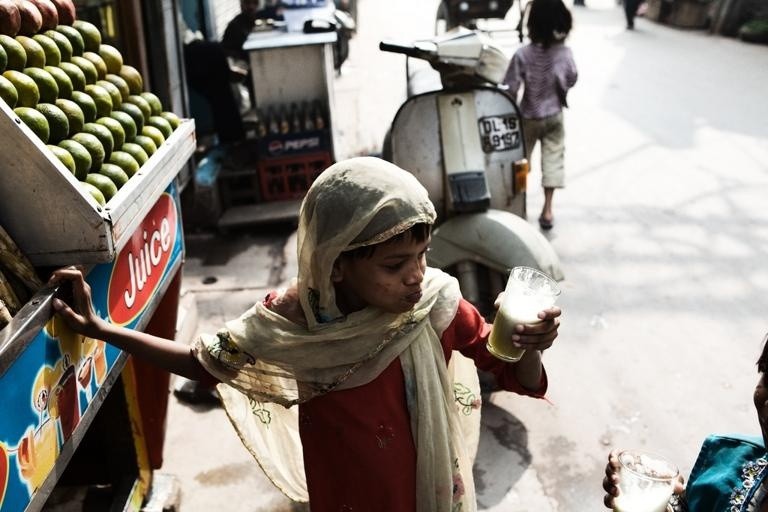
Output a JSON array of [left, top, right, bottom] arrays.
[[611, 450, 680, 512], [486, 266, 561, 362], [21, 342, 108, 501]]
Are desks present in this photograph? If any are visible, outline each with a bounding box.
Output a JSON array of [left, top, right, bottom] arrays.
[[240, 21, 341, 162]]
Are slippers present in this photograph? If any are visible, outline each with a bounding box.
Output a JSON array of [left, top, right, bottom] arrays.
[[539, 213, 554, 231]]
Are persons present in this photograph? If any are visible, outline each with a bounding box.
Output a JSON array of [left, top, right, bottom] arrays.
[[602, 329, 765, 511], [54, 156, 561, 512], [499, 1, 577, 227]]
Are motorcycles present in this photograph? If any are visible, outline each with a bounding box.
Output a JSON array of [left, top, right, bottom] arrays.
[[378, 23, 565, 324]]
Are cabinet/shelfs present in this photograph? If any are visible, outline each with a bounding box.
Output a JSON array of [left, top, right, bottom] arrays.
[[0, 176, 186, 512]]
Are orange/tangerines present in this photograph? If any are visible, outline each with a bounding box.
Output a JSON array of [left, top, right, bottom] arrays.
[[0, 0, 179, 207]]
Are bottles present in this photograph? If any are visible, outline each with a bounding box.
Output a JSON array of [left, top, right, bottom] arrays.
[[255, 98, 323, 135]]
